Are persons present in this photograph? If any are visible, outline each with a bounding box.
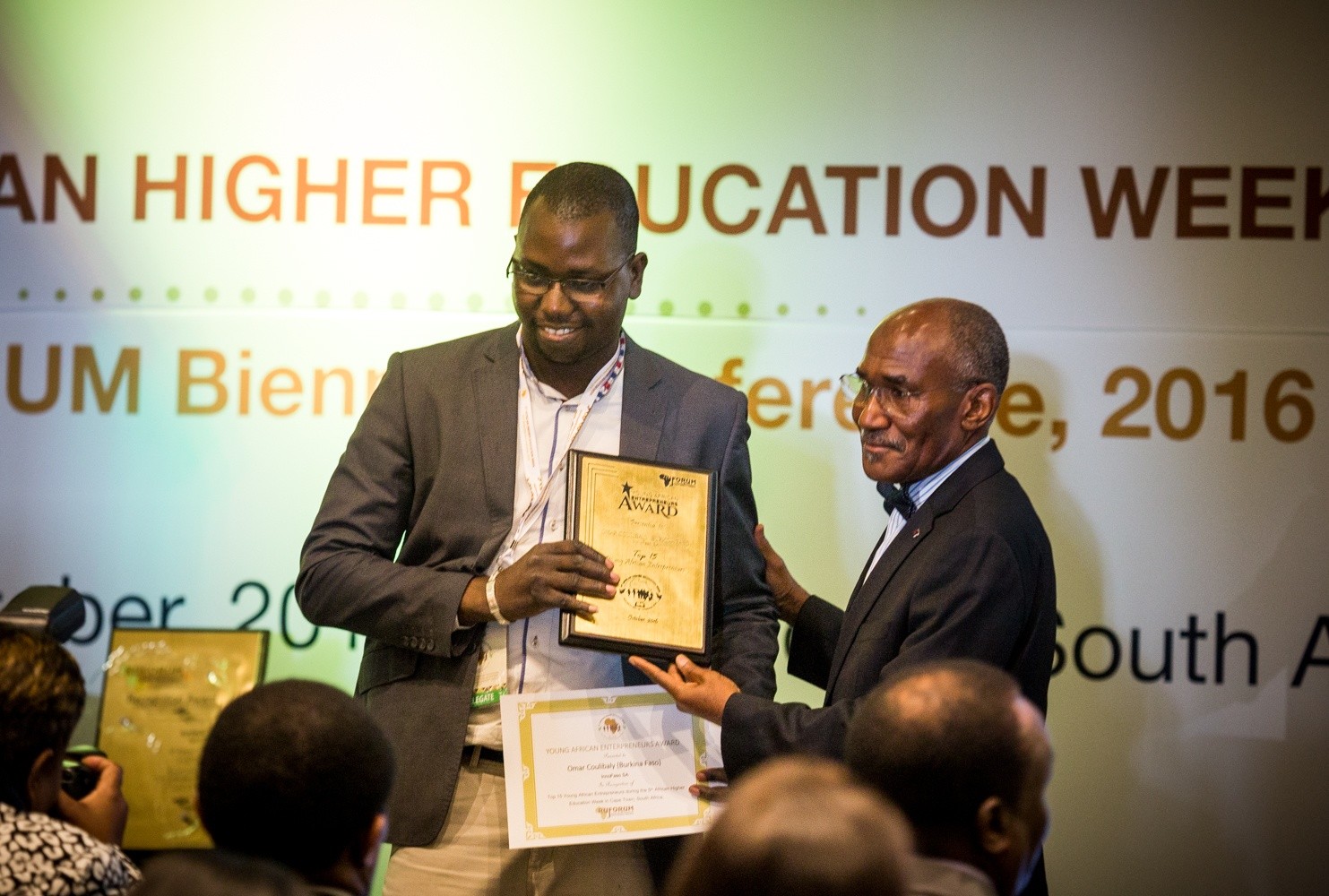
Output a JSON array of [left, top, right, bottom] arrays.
[[297, 164, 778, 896], [629, 294, 1056, 896], [663, 749, 919, 896], [0, 624, 147, 896], [840, 651, 1057, 896], [125, 679, 394, 895]]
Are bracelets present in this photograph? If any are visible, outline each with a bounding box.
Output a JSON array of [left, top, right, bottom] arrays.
[[484, 573, 514, 626]]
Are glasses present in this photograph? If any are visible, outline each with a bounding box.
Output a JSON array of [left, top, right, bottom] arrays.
[[840, 371, 980, 414], [505, 250, 635, 303]]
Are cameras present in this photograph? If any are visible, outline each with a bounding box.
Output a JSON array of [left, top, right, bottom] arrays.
[[54, 741, 109, 827]]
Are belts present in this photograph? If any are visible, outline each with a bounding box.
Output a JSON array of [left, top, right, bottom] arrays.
[[463, 736, 503, 763]]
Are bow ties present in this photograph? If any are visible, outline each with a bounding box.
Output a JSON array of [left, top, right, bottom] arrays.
[[877, 481, 916, 520]]
